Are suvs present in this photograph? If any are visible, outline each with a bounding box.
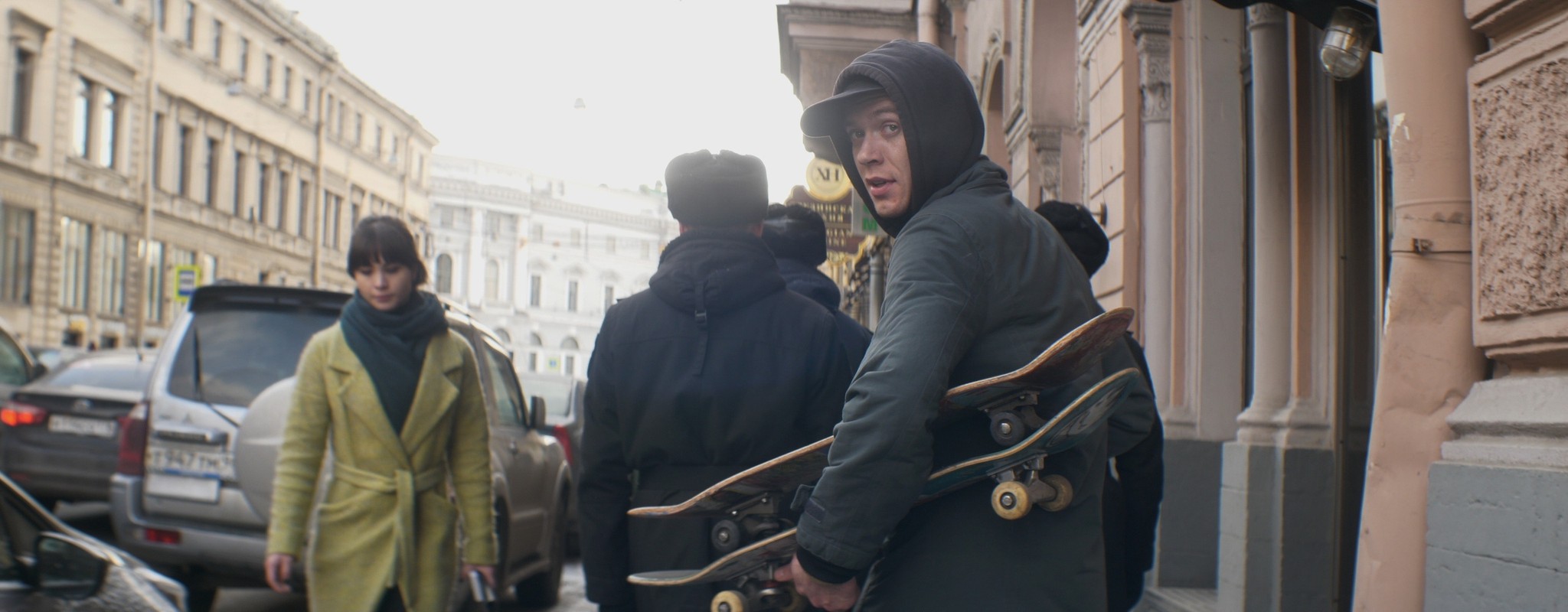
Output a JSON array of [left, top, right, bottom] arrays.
[[106, 277, 576, 612]]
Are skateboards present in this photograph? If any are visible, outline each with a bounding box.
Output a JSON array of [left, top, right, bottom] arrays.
[[626, 364, 1143, 612], [626, 306, 1136, 559]]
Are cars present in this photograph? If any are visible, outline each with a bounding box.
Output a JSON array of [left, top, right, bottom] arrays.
[[514, 369, 585, 560], [0, 312, 196, 612]]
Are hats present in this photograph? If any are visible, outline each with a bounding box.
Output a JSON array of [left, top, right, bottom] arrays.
[[1035, 200, 1110, 279], [666, 149, 770, 228], [763, 204, 827, 265], [800, 76, 885, 138]]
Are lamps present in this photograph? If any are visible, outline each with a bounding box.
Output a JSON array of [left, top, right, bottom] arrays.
[[1319, 6, 1381, 80]]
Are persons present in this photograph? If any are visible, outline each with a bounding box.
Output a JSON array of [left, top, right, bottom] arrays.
[[263, 213, 499, 612], [579, 148, 875, 611], [1014, 199, 1165, 612], [775, 38, 1158, 612]]
